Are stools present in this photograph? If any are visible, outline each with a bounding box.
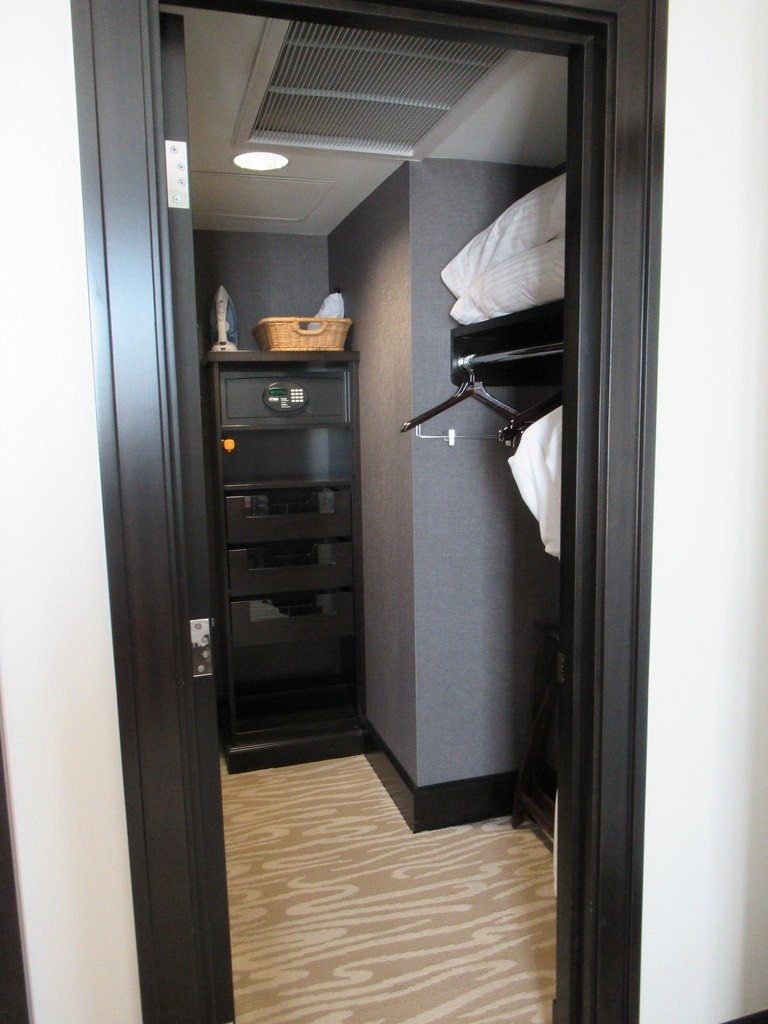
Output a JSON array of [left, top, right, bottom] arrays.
[[512, 619, 561, 845]]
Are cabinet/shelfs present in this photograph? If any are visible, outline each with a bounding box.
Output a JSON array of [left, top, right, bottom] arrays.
[[207, 350, 378, 774]]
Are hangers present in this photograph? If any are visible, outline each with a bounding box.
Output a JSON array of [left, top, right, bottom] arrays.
[[499, 389, 563, 447], [402, 355, 524, 446]]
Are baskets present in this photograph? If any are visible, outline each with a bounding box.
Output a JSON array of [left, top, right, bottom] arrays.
[[251, 316, 352, 351]]
